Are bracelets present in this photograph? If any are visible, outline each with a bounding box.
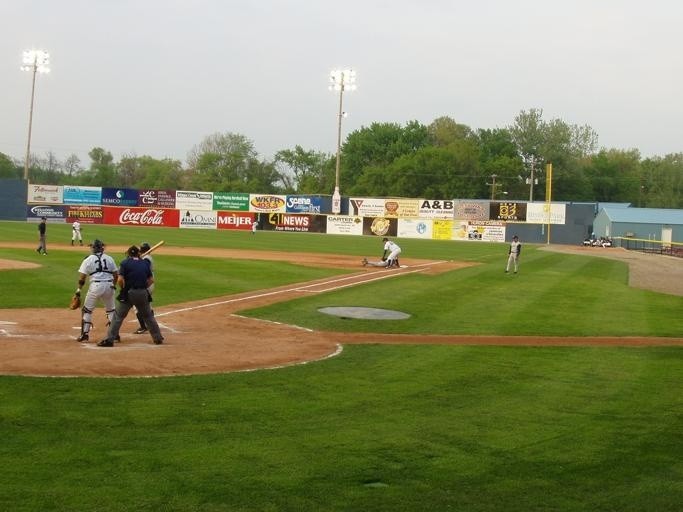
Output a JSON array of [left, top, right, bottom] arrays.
[[77, 288, 81, 293]]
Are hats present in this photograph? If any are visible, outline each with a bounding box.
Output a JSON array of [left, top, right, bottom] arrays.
[[42, 216, 47, 220]]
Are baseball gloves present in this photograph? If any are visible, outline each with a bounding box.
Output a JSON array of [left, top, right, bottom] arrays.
[[69, 292, 80, 311]]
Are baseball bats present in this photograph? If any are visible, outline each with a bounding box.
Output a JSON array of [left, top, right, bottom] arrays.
[[140, 241, 165, 258]]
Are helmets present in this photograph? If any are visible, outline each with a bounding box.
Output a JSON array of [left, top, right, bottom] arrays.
[[91, 238, 104, 253], [141, 241, 151, 253]]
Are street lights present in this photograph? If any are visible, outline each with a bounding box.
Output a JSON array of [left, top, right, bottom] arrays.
[[326, 68, 355, 197]]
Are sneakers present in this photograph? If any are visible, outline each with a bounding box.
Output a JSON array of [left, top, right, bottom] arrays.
[[136, 327, 146, 334], [37, 248, 48, 255], [97, 340, 114, 347], [76, 335, 89, 342]]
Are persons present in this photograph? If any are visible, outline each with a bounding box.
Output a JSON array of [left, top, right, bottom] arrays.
[[361, 258, 389, 267], [73, 239, 120, 343], [97, 245, 164, 347], [71, 218, 83, 246], [599, 236, 613, 248], [381, 237, 402, 269], [251, 220, 259, 234], [132, 242, 154, 334], [467, 221, 473, 239], [504, 234, 521, 274], [34, 216, 48, 255]]
